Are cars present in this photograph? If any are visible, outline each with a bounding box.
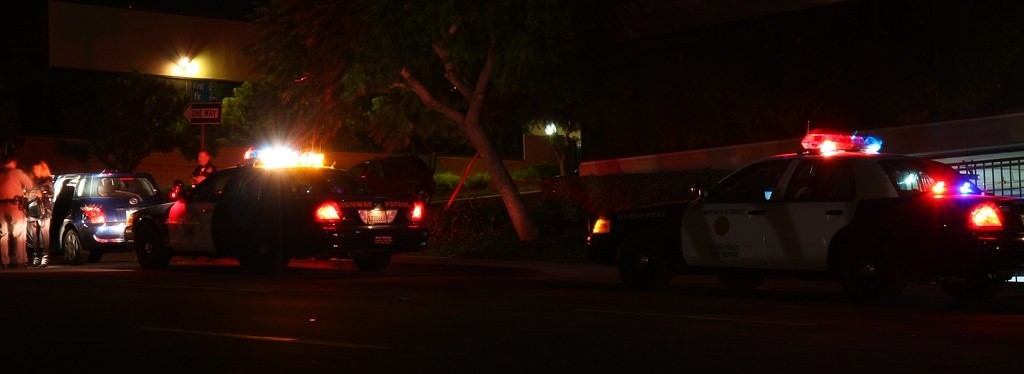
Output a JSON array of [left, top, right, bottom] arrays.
[[347, 157, 439, 208], [21, 169, 167, 265], [584, 131, 1024, 309], [129, 166, 431, 279]]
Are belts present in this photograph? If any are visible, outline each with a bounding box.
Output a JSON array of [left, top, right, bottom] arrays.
[[1, 199, 15, 203]]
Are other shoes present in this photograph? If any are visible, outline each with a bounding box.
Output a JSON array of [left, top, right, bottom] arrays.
[[32, 257, 40, 267], [40, 255, 50, 266], [3, 264, 10, 269]]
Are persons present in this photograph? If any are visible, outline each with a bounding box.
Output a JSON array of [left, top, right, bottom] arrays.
[[190, 149, 219, 188], [0, 159, 54, 267]]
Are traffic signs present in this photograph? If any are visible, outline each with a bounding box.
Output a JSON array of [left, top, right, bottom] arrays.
[[180, 101, 222, 125]]
